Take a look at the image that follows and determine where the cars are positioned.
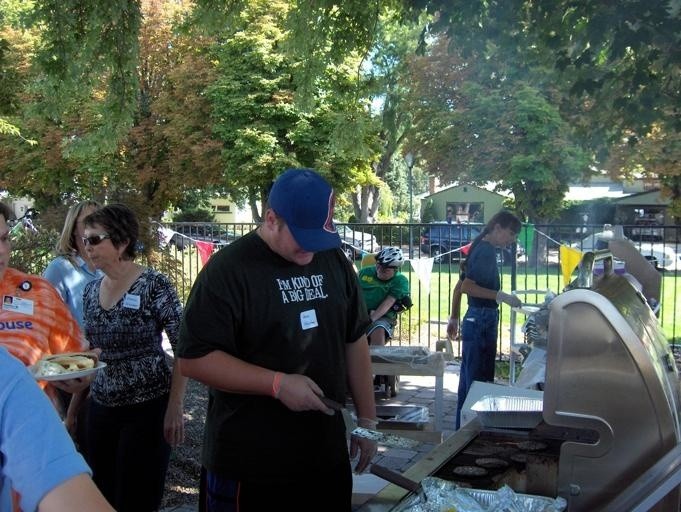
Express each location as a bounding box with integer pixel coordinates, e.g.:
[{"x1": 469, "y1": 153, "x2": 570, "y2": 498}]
[
  {"x1": 331, "y1": 220, "x2": 380, "y2": 261},
  {"x1": 629, "y1": 218, "x2": 664, "y2": 240},
  {"x1": 559, "y1": 232, "x2": 675, "y2": 269},
  {"x1": 162, "y1": 220, "x2": 241, "y2": 249}
]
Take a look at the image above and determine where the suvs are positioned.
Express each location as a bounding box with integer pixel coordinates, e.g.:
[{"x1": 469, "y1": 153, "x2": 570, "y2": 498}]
[{"x1": 419, "y1": 221, "x2": 523, "y2": 265}]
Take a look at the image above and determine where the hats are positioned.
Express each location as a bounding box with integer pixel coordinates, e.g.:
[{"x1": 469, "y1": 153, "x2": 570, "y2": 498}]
[{"x1": 267, "y1": 168, "x2": 343, "y2": 253}]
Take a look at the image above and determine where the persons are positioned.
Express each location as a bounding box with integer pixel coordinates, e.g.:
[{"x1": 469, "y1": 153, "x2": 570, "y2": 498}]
[
  {"x1": 179, "y1": 167, "x2": 382, "y2": 512},
  {"x1": 63, "y1": 204, "x2": 190, "y2": 512},
  {"x1": 39, "y1": 199, "x2": 103, "y2": 423},
  {"x1": 0, "y1": 344, "x2": 116, "y2": 512},
  {"x1": 357, "y1": 246, "x2": 411, "y2": 391},
  {"x1": 447, "y1": 210, "x2": 522, "y2": 432},
  {"x1": 0, "y1": 198, "x2": 104, "y2": 396}
]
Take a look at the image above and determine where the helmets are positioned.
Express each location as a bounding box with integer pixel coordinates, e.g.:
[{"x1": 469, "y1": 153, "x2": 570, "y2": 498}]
[{"x1": 374, "y1": 248, "x2": 404, "y2": 269}]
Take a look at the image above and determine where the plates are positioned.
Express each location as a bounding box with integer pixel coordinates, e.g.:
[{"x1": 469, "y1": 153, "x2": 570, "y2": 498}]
[{"x1": 27, "y1": 360, "x2": 108, "y2": 382}]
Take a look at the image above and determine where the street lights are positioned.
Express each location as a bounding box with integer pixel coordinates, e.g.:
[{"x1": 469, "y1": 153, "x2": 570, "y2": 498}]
[{"x1": 404, "y1": 152, "x2": 416, "y2": 260}]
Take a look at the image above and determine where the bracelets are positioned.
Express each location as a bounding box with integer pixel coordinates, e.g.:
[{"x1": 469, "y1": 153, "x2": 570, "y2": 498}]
[
  {"x1": 357, "y1": 415, "x2": 380, "y2": 426},
  {"x1": 271, "y1": 371, "x2": 283, "y2": 399}
]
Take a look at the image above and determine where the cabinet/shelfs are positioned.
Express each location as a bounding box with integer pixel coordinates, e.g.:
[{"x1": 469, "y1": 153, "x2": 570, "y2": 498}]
[{"x1": 509, "y1": 290, "x2": 557, "y2": 388}]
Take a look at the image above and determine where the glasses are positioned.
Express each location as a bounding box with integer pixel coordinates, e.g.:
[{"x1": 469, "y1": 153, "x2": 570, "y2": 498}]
[{"x1": 80, "y1": 233, "x2": 113, "y2": 246}]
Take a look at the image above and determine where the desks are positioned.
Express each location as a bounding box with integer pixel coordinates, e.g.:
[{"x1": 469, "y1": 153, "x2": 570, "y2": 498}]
[{"x1": 367, "y1": 338, "x2": 455, "y2": 435}]
